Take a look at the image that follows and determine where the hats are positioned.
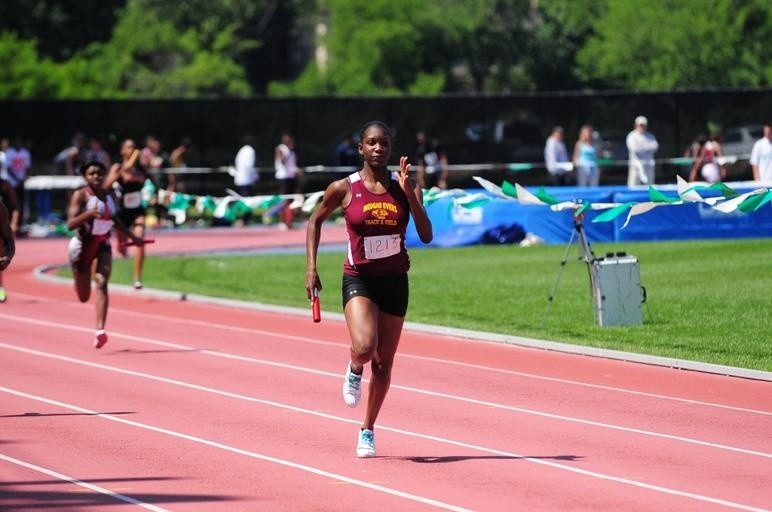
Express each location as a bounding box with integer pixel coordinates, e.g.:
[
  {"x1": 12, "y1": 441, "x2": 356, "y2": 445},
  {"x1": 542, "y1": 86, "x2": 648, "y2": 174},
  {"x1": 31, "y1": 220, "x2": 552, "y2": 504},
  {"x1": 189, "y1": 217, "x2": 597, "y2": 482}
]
[{"x1": 635, "y1": 115, "x2": 648, "y2": 125}]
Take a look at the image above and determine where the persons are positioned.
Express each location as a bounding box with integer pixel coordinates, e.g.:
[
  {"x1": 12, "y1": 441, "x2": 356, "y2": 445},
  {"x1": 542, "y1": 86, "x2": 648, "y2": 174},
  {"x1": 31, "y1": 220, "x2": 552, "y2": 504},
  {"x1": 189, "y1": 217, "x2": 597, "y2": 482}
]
[
  {"x1": 1, "y1": 160, "x2": 20, "y2": 302},
  {"x1": 0, "y1": 138, "x2": 190, "y2": 239},
  {"x1": 1, "y1": 201, "x2": 14, "y2": 272},
  {"x1": 66, "y1": 161, "x2": 143, "y2": 349},
  {"x1": 544, "y1": 116, "x2": 771, "y2": 186},
  {"x1": 102, "y1": 139, "x2": 158, "y2": 289},
  {"x1": 235, "y1": 130, "x2": 448, "y2": 189},
  {"x1": 304, "y1": 121, "x2": 434, "y2": 457}
]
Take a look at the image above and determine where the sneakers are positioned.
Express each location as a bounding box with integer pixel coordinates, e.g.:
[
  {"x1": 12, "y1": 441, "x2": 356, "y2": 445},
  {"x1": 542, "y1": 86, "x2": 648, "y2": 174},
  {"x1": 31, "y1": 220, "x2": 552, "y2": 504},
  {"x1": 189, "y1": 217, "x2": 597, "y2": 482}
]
[
  {"x1": 356, "y1": 427, "x2": 376, "y2": 459},
  {"x1": 134, "y1": 281, "x2": 142, "y2": 289},
  {"x1": 0, "y1": 287, "x2": 7, "y2": 302},
  {"x1": 341, "y1": 359, "x2": 363, "y2": 409},
  {"x1": 94, "y1": 330, "x2": 108, "y2": 349}
]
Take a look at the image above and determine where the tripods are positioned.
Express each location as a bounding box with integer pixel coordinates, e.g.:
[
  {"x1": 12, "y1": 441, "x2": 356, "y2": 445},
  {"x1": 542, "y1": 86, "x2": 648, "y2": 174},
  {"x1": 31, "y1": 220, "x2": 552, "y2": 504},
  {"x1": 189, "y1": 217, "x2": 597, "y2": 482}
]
[{"x1": 537, "y1": 222, "x2": 596, "y2": 331}]
